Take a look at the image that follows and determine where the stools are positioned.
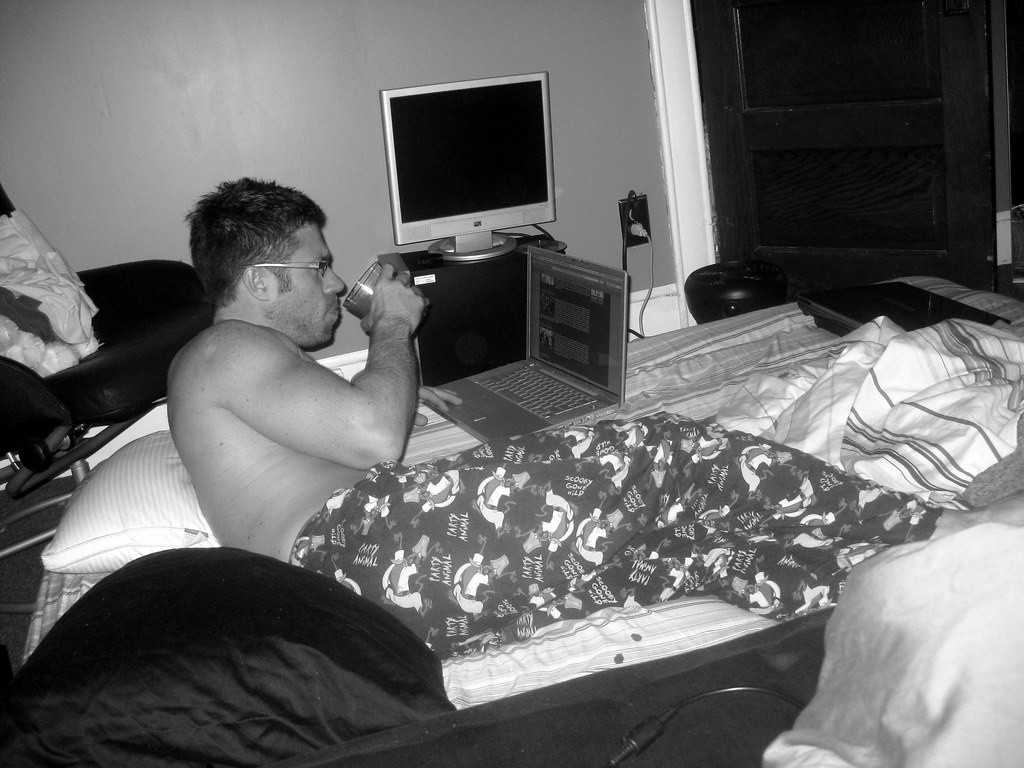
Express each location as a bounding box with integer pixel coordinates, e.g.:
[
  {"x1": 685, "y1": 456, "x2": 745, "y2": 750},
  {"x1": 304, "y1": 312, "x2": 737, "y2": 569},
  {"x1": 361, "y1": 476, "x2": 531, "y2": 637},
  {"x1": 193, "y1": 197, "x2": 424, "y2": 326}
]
[{"x1": 684, "y1": 262, "x2": 788, "y2": 324}]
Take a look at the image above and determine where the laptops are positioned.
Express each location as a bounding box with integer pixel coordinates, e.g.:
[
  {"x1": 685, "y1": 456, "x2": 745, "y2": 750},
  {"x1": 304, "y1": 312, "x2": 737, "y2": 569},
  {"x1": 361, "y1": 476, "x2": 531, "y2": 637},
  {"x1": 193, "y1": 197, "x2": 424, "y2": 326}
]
[
  {"x1": 794, "y1": 280, "x2": 1012, "y2": 337},
  {"x1": 419, "y1": 245, "x2": 631, "y2": 447}
]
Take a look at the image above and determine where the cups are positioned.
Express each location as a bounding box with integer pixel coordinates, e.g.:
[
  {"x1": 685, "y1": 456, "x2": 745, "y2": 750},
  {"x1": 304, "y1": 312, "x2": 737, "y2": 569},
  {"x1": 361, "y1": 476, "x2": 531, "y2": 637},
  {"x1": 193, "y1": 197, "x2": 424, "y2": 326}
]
[{"x1": 343, "y1": 263, "x2": 382, "y2": 319}]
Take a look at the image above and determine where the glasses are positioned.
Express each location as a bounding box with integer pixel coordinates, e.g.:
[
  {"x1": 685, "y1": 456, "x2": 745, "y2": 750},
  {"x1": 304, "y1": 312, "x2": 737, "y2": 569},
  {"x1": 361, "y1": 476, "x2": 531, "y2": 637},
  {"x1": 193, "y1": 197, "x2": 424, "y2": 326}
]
[{"x1": 230, "y1": 257, "x2": 337, "y2": 278}]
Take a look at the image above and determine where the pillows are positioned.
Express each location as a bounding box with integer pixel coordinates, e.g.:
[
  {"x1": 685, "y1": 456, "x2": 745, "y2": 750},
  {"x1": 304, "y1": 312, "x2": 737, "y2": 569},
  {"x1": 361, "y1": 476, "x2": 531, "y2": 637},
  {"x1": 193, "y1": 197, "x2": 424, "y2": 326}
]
[{"x1": 41, "y1": 432, "x2": 220, "y2": 575}]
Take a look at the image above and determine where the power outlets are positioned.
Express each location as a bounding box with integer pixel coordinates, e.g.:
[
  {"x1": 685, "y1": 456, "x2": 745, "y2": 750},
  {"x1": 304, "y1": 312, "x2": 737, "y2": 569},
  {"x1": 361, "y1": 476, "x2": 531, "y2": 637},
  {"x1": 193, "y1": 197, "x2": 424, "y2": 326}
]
[{"x1": 618, "y1": 194, "x2": 651, "y2": 248}]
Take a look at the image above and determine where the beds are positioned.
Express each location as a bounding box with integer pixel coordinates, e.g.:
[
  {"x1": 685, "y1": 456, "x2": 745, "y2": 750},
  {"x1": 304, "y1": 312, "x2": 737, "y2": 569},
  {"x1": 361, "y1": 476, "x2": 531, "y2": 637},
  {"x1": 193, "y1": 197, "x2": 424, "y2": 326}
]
[{"x1": 22, "y1": 270, "x2": 1024, "y2": 768}]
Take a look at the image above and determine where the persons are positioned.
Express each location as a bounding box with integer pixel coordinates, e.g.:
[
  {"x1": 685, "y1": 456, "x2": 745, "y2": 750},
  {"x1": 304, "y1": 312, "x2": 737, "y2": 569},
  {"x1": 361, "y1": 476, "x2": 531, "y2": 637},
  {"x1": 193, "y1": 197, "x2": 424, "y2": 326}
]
[{"x1": 167, "y1": 173, "x2": 1024, "y2": 661}]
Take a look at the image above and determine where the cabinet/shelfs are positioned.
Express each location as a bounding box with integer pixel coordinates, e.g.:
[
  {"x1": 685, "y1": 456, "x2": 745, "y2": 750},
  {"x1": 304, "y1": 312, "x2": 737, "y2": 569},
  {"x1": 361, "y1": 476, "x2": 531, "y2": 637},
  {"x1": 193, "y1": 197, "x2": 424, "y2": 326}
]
[{"x1": 378, "y1": 234, "x2": 567, "y2": 390}]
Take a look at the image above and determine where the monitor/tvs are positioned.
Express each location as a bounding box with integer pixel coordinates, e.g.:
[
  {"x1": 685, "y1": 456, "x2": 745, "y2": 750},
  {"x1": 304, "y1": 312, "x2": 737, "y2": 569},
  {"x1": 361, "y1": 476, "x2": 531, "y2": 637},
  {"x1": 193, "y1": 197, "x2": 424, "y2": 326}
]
[{"x1": 379, "y1": 70, "x2": 556, "y2": 262}]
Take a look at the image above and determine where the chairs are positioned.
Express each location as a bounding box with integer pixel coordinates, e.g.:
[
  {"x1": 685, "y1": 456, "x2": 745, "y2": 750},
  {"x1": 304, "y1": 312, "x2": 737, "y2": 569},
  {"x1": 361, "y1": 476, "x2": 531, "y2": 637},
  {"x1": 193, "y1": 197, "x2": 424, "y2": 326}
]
[{"x1": 0, "y1": 260, "x2": 213, "y2": 616}]
